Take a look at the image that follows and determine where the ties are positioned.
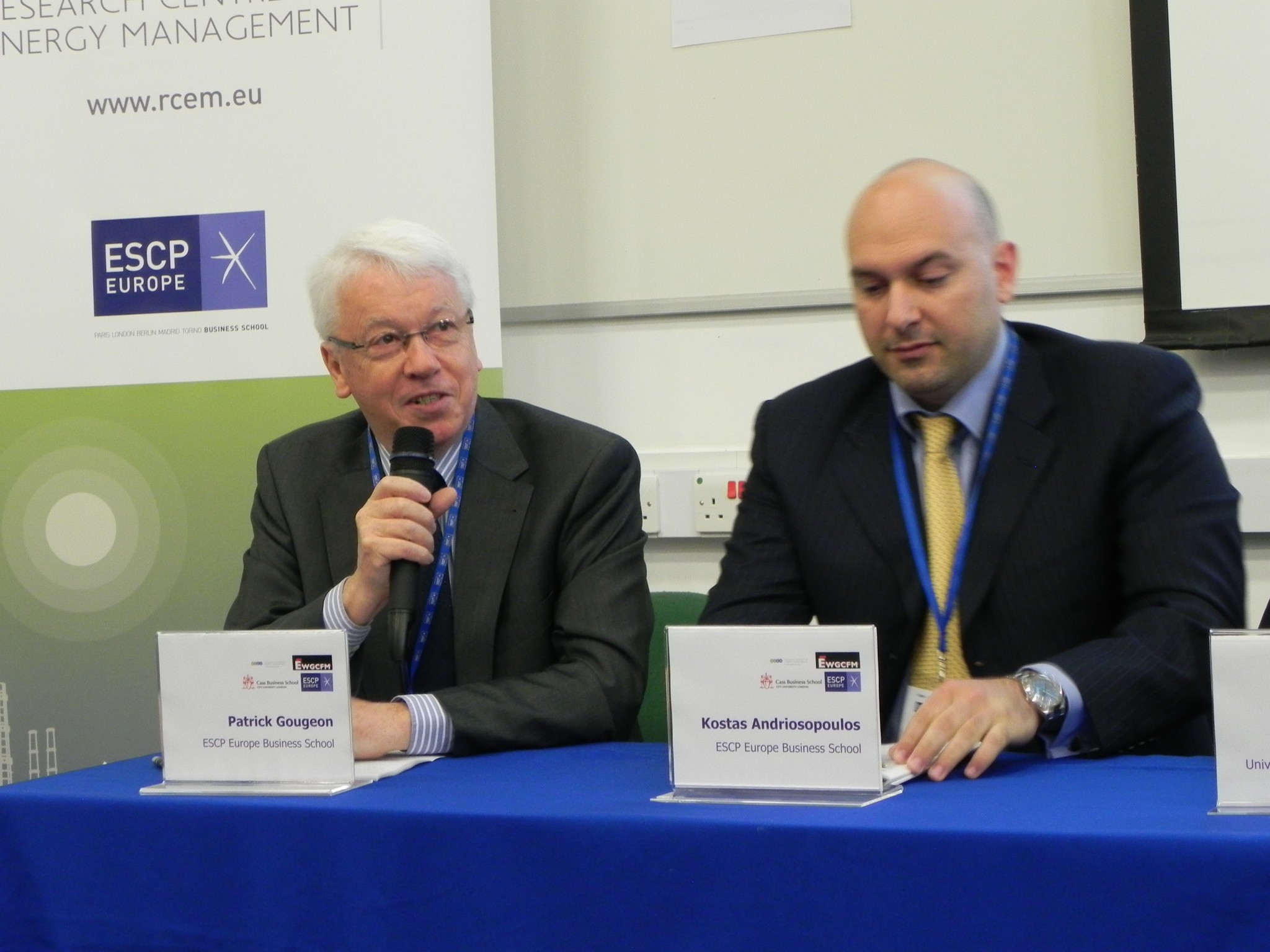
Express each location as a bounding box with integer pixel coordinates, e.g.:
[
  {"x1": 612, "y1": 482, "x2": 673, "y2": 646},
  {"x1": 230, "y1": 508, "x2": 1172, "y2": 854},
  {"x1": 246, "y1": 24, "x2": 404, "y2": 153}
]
[
  {"x1": 909, "y1": 412, "x2": 972, "y2": 690},
  {"x1": 402, "y1": 518, "x2": 453, "y2": 695}
]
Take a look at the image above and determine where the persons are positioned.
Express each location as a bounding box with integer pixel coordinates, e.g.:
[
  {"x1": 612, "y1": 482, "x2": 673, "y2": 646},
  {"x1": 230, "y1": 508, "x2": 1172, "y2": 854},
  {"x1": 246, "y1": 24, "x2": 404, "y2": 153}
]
[
  {"x1": 689, "y1": 159, "x2": 1249, "y2": 780},
  {"x1": 222, "y1": 222, "x2": 655, "y2": 758}
]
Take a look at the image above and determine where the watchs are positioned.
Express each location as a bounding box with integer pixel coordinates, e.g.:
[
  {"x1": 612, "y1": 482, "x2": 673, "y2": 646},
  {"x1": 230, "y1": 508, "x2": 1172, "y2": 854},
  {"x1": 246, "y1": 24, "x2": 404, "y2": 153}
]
[{"x1": 1008, "y1": 671, "x2": 1068, "y2": 750}]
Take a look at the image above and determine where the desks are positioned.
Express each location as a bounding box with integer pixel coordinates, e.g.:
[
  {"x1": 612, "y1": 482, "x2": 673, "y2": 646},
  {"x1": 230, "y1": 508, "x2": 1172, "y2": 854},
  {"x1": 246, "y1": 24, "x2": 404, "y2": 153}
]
[{"x1": 0, "y1": 733, "x2": 1270, "y2": 952}]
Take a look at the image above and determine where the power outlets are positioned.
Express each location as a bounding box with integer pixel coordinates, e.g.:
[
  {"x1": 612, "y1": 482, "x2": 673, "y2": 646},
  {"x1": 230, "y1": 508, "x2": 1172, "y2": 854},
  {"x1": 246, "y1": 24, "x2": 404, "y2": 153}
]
[
  {"x1": 692, "y1": 468, "x2": 751, "y2": 533},
  {"x1": 637, "y1": 470, "x2": 661, "y2": 537}
]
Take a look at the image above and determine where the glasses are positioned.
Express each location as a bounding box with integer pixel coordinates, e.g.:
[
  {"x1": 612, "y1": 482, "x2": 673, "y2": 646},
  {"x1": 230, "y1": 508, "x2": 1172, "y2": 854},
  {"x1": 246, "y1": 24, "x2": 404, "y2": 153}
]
[{"x1": 328, "y1": 308, "x2": 474, "y2": 362}]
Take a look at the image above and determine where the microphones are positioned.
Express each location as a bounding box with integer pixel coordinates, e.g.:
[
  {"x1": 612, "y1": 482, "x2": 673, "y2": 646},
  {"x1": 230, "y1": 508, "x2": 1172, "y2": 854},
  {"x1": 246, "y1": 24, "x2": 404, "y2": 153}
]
[{"x1": 390, "y1": 425, "x2": 435, "y2": 659}]
